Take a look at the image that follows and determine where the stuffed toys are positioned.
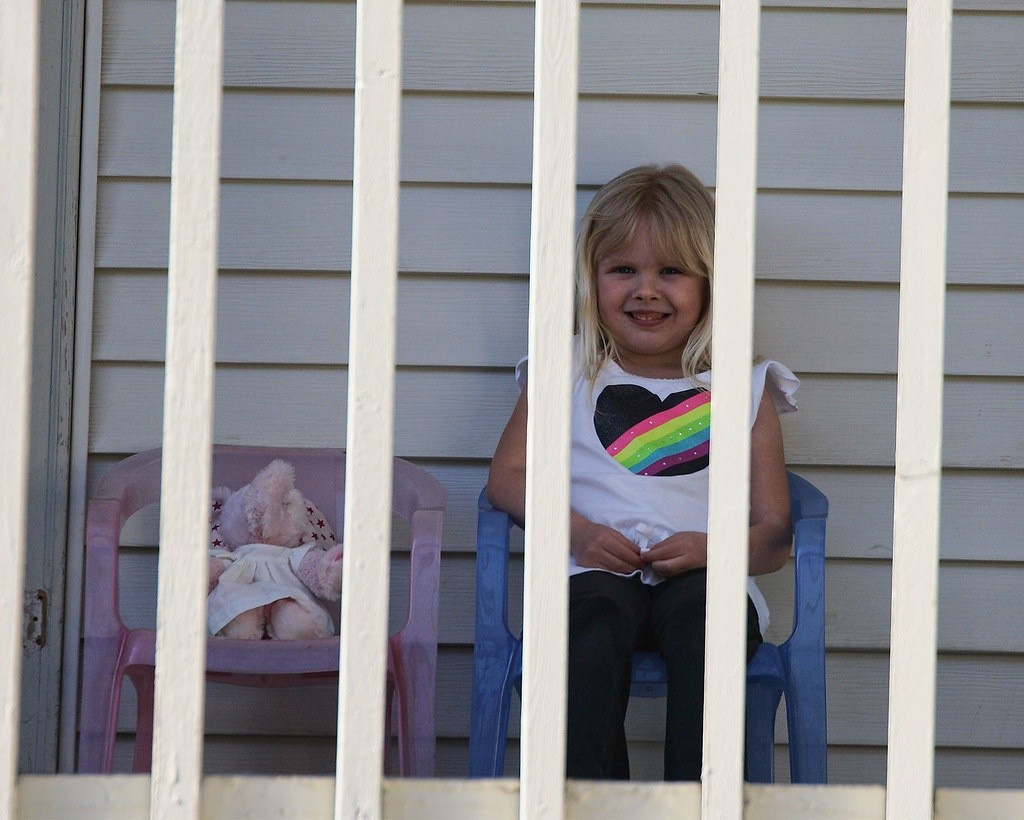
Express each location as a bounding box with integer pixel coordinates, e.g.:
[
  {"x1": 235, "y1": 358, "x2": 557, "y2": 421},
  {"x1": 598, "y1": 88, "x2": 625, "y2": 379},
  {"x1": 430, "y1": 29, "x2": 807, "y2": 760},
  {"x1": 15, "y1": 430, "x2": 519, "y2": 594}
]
[{"x1": 206, "y1": 456, "x2": 343, "y2": 641}]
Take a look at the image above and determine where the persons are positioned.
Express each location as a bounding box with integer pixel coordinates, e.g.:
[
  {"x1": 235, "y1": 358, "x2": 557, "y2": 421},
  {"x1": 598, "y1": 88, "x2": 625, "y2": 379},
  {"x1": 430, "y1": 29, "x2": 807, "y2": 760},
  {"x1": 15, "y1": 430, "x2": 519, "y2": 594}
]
[{"x1": 482, "y1": 162, "x2": 801, "y2": 781}]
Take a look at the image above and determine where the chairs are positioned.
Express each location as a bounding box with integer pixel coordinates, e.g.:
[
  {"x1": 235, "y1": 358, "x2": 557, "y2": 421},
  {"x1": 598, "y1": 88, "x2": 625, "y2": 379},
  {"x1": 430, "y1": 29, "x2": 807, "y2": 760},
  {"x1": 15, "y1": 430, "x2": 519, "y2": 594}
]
[
  {"x1": 76, "y1": 444, "x2": 447, "y2": 780},
  {"x1": 468, "y1": 467, "x2": 830, "y2": 786}
]
